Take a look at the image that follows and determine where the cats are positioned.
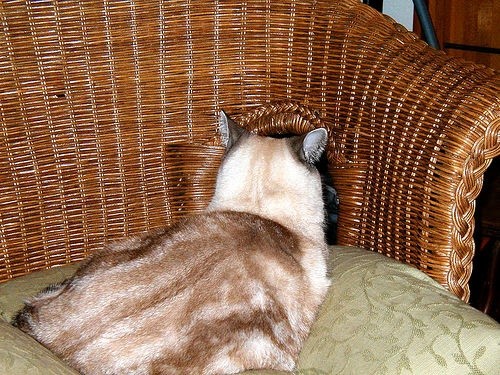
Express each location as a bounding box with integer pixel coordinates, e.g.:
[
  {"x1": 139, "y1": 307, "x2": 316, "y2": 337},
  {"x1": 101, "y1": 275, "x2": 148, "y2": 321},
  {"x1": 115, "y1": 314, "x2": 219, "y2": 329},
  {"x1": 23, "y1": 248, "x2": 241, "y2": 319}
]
[{"x1": 15, "y1": 110, "x2": 330, "y2": 375}]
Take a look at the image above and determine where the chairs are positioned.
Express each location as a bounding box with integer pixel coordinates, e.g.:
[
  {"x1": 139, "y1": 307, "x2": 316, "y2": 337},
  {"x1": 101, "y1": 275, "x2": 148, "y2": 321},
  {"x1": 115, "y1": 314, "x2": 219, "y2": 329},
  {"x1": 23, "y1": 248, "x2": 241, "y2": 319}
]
[{"x1": 0, "y1": 0, "x2": 500, "y2": 306}]
